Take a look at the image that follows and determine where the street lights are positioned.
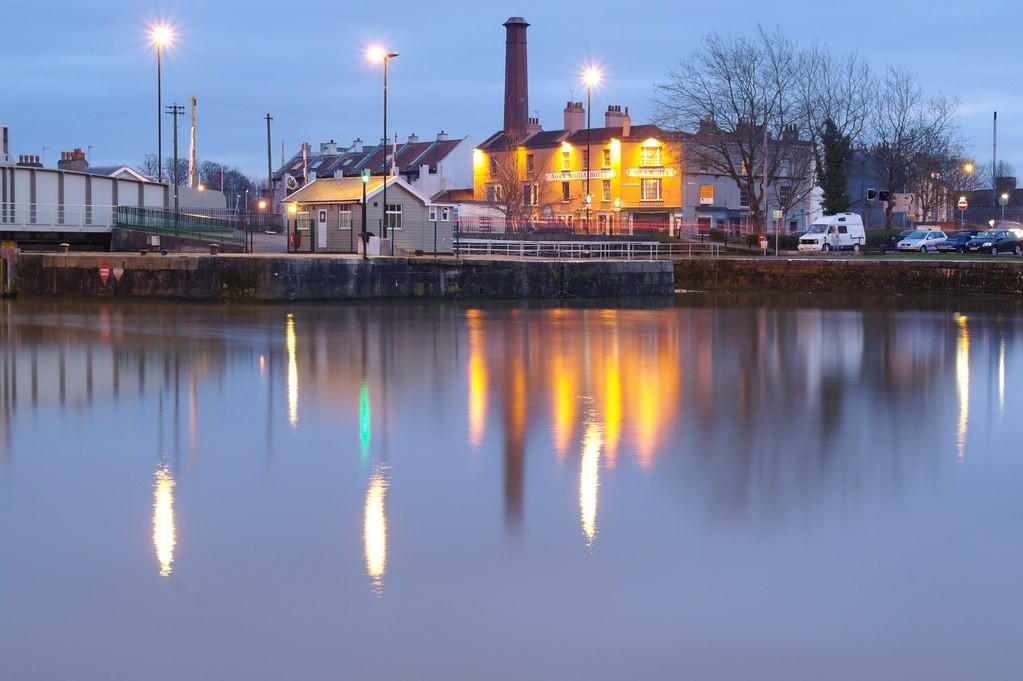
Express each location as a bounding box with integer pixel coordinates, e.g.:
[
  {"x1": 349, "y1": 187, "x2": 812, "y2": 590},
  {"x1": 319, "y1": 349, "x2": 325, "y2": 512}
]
[
  {"x1": 155, "y1": 27, "x2": 170, "y2": 181},
  {"x1": 362, "y1": 171, "x2": 367, "y2": 259},
  {"x1": 963, "y1": 163, "x2": 971, "y2": 196},
  {"x1": 369, "y1": 49, "x2": 399, "y2": 237},
  {"x1": 586, "y1": 68, "x2": 592, "y2": 235}
]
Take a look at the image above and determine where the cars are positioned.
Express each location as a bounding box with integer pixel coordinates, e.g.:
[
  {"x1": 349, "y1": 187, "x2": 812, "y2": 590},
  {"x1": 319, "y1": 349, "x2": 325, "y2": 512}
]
[
  {"x1": 959, "y1": 230, "x2": 984, "y2": 236},
  {"x1": 892, "y1": 230, "x2": 923, "y2": 249},
  {"x1": 966, "y1": 230, "x2": 1021, "y2": 256},
  {"x1": 936, "y1": 234, "x2": 975, "y2": 254},
  {"x1": 897, "y1": 231, "x2": 948, "y2": 254}
]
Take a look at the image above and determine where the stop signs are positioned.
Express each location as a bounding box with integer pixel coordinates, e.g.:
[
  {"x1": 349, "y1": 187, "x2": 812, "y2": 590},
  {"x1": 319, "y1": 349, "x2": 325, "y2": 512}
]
[{"x1": 958, "y1": 200, "x2": 968, "y2": 210}]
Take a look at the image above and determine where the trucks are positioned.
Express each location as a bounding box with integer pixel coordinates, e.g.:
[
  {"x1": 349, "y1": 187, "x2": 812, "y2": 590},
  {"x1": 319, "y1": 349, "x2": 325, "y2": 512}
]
[{"x1": 798, "y1": 212, "x2": 866, "y2": 251}]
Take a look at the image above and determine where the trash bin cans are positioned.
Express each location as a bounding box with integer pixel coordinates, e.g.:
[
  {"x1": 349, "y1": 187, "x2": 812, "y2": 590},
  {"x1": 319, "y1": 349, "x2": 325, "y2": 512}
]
[
  {"x1": 209, "y1": 244, "x2": 219, "y2": 255},
  {"x1": 880, "y1": 244, "x2": 887, "y2": 255}
]
